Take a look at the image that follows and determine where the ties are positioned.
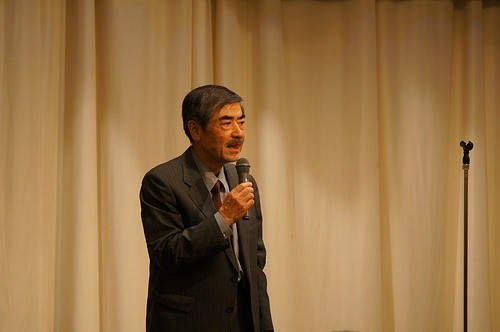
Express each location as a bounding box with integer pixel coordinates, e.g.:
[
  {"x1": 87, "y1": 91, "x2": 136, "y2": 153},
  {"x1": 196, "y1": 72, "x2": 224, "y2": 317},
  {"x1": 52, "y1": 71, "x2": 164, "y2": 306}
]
[{"x1": 209, "y1": 179, "x2": 241, "y2": 279}]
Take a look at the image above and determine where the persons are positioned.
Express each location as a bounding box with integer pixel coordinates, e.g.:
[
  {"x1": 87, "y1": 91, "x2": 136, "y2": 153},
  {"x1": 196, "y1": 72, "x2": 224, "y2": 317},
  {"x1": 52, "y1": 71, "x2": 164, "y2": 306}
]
[{"x1": 139, "y1": 85, "x2": 274, "y2": 332}]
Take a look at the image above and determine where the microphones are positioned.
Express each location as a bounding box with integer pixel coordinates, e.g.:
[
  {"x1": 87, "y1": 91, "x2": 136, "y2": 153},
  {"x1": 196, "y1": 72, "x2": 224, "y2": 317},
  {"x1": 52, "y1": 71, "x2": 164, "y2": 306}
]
[{"x1": 234, "y1": 158, "x2": 251, "y2": 219}]
